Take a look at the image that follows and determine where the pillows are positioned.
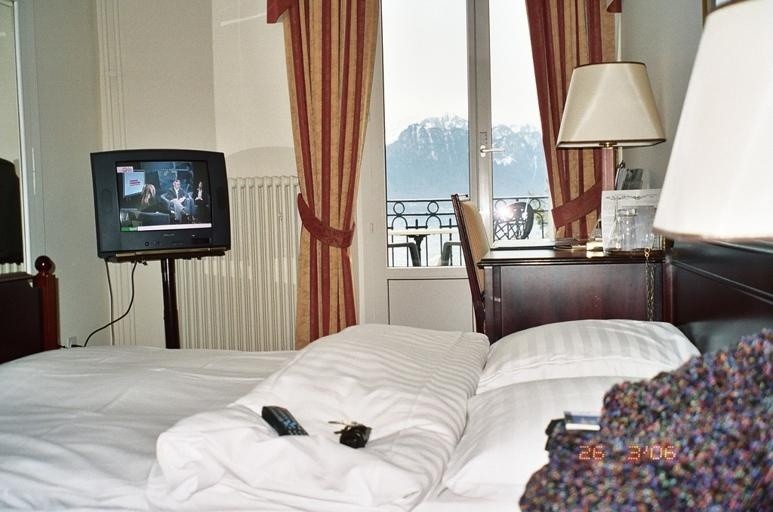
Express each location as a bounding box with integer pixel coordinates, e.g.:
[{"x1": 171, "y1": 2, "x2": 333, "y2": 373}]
[
  {"x1": 475, "y1": 317, "x2": 703, "y2": 396},
  {"x1": 439, "y1": 377, "x2": 654, "y2": 504}
]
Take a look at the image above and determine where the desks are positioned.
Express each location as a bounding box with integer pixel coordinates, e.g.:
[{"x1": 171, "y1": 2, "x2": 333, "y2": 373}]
[
  {"x1": 390, "y1": 228, "x2": 456, "y2": 266},
  {"x1": 476, "y1": 236, "x2": 664, "y2": 345}
]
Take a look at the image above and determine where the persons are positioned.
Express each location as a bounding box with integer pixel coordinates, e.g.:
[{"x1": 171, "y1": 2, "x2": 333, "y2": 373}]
[
  {"x1": 137, "y1": 184, "x2": 168, "y2": 214},
  {"x1": 191, "y1": 180, "x2": 208, "y2": 224},
  {"x1": 167, "y1": 180, "x2": 189, "y2": 224}
]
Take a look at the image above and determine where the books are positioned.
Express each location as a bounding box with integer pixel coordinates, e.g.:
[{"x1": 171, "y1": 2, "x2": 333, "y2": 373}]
[
  {"x1": 591, "y1": 169, "x2": 649, "y2": 238},
  {"x1": 601, "y1": 188, "x2": 665, "y2": 253}
]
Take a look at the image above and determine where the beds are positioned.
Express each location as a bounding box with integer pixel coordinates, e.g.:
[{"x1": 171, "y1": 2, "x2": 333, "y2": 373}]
[{"x1": 0, "y1": 238, "x2": 773, "y2": 512}]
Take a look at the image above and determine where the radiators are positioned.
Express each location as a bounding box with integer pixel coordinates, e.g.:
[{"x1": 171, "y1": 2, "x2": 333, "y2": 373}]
[{"x1": 177, "y1": 176, "x2": 302, "y2": 352}]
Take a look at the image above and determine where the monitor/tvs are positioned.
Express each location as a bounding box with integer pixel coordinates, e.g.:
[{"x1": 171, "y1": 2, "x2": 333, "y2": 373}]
[{"x1": 90, "y1": 149, "x2": 231, "y2": 263}]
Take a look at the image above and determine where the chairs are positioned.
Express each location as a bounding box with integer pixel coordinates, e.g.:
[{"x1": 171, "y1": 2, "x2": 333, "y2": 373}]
[{"x1": 439, "y1": 191, "x2": 533, "y2": 332}]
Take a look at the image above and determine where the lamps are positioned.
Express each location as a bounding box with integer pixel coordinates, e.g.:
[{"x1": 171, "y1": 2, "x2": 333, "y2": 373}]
[{"x1": 553, "y1": 60, "x2": 669, "y2": 251}]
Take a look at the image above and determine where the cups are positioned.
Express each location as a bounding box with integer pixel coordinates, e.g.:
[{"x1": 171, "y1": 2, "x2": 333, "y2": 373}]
[{"x1": 616, "y1": 214, "x2": 636, "y2": 250}]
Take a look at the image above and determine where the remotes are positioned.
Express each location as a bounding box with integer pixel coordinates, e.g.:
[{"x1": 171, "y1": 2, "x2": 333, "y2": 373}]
[{"x1": 262, "y1": 406, "x2": 308, "y2": 436}]
